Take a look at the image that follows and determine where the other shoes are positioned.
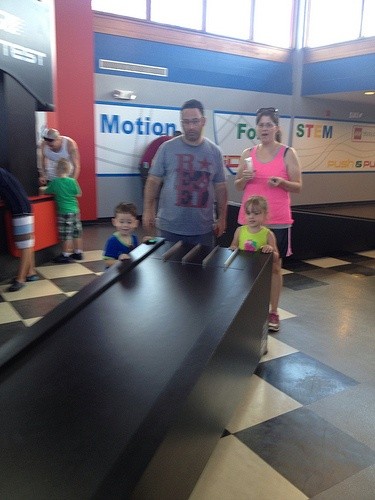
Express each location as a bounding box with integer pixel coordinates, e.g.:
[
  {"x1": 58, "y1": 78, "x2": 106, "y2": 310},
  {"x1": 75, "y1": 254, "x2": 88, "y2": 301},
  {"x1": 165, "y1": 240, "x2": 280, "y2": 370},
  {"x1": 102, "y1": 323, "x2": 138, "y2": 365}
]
[
  {"x1": 26, "y1": 275, "x2": 39, "y2": 281},
  {"x1": 7, "y1": 282, "x2": 26, "y2": 291}
]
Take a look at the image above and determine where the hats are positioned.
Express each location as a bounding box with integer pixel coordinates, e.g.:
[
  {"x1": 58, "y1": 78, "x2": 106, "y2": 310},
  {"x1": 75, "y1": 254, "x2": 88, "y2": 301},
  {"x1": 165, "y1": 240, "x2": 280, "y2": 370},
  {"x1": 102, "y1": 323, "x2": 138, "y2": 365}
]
[{"x1": 42, "y1": 128, "x2": 59, "y2": 139}]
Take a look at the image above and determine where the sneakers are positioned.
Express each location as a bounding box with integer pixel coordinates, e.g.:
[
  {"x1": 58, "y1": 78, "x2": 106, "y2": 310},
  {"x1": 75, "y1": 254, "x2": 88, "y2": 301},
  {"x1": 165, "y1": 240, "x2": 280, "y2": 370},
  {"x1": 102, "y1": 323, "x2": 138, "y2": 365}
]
[
  {"x1": 73, "y1": 253, "x2": 82, "y2": 260},
  {"x1": 55, "y1": 254, "x2": 73, "y2": 262},
  {"x1": 268, "y1": 311, "x2": 280, "y2": 331}
]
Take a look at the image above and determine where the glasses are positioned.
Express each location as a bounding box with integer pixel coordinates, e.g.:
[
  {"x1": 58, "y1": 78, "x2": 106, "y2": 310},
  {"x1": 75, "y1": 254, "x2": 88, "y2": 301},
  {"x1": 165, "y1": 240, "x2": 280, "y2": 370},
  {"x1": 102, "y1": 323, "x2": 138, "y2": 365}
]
[{"x1": 256, "y1": 107, "x2": 278, "y2": 115}]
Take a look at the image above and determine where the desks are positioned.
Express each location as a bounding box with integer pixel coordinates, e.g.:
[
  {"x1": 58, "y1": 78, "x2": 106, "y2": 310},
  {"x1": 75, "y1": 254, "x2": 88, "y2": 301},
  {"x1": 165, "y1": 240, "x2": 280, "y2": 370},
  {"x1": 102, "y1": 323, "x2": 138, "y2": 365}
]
[
  {"x1": 7, "y1": 193, "x2": 60, "y2": 257},
  {"x1": 0, "y1": 236, "x2": 274, "y2": 500}
]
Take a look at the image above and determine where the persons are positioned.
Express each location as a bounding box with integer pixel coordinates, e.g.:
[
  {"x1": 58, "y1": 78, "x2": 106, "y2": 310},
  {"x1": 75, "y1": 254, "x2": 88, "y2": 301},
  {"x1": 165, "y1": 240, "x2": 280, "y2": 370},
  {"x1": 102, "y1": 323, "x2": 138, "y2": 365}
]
[
  {"x1": 235, "y1": 107, "x2": 303, "y2": 332},
  {"x1": 229, "y1": 196, "x2": 280, "y2": 253},
  {"x1": 44, "y1": 159, "x2": 83, "y2": 263},
  {"x1": 40, "y1": 128, "x2": 80, "y2": 186},
  {"x1": 104, "y1": 203, "x2": 150, "y2": 270},
  {"x1": 139, "y1": 131, "x2": 182, "y2": 201},
  {"x1": 0, "y1": 168, "x2": 39, "y2": 291},
  {"x1": 143, "y1": 101, "x2": 229, "y2": 247}
]
